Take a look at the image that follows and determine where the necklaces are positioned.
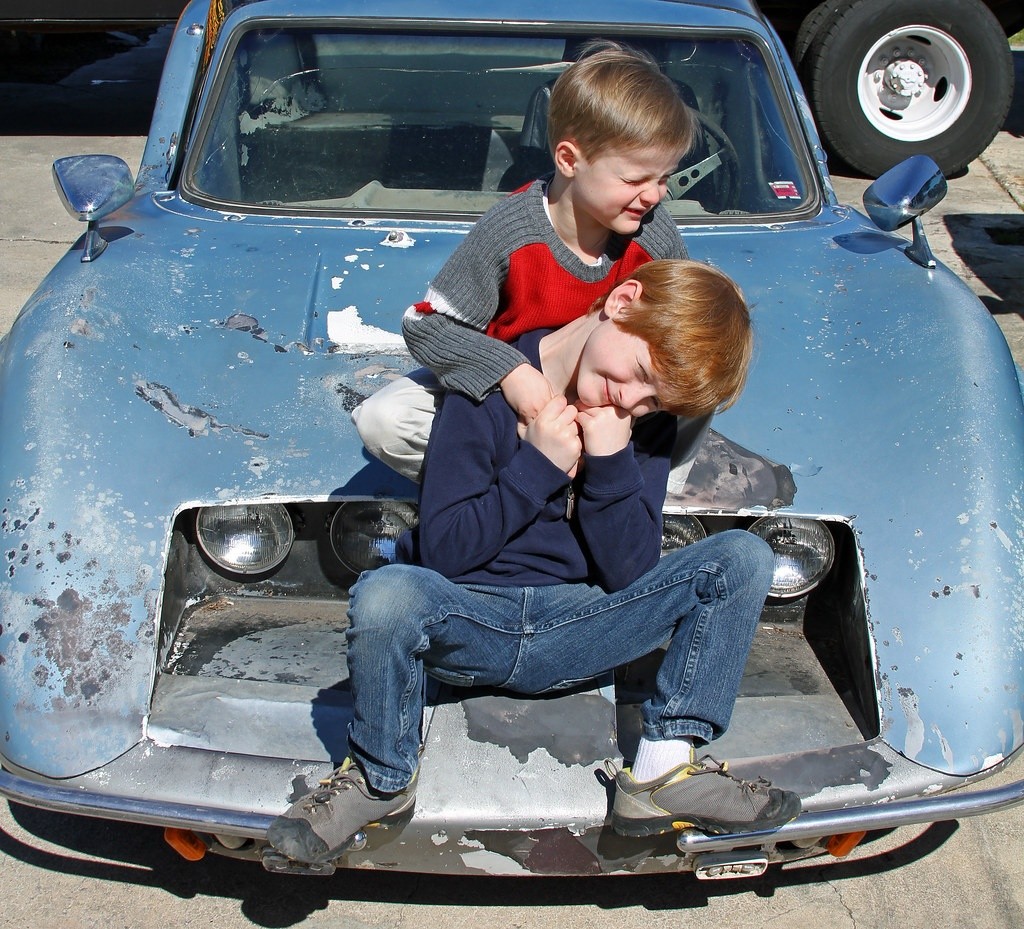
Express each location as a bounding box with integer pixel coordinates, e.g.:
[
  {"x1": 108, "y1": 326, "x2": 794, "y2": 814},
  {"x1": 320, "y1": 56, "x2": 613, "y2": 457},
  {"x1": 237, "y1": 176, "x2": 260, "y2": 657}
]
[{"x1": 567, "y1": 485, "x2": 574, "y2": 519}]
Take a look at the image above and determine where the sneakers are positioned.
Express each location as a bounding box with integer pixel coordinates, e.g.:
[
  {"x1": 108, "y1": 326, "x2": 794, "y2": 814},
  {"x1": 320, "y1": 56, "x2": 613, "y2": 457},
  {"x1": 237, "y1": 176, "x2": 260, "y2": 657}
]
[
  {"x1": 268, "y1": 756, "x2": 421, "y2": 863},
  {"x1": 604, "y1": 748, "x2": 802, "y2": 838}
]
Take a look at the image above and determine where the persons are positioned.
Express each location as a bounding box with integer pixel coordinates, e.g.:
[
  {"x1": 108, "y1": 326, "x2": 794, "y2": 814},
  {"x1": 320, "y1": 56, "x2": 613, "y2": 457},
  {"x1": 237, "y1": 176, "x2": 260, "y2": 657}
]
[
  {"x1": 265, "y1": 259, "x2": 800, "y2": 865},
  {"x1": 349, "y1": 39, "x2": 691, "y2": 487}
]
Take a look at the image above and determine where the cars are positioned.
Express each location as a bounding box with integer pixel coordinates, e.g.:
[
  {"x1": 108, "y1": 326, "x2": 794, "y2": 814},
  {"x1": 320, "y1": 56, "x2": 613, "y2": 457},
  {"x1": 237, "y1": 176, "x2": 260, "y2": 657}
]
[
  {"x1": 0, "y1": 0, "x2": 1024, "y2": 185},
  {"x1": 0, "y1": 0, "x2": 1021, "y2": 896}
]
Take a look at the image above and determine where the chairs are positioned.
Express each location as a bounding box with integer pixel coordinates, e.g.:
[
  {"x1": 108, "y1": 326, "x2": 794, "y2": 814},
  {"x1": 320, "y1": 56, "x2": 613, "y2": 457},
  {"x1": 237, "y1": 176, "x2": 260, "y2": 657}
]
[{"x1": 513, "y1": 37, "x2": 714, "y2": 209}]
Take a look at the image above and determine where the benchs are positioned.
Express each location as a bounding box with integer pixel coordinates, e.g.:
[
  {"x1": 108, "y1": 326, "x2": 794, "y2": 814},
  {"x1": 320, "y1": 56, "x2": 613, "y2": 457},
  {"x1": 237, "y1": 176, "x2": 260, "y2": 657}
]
[{"x1": 262, "y1": 55, "x2": 561, "y2": 179}]
